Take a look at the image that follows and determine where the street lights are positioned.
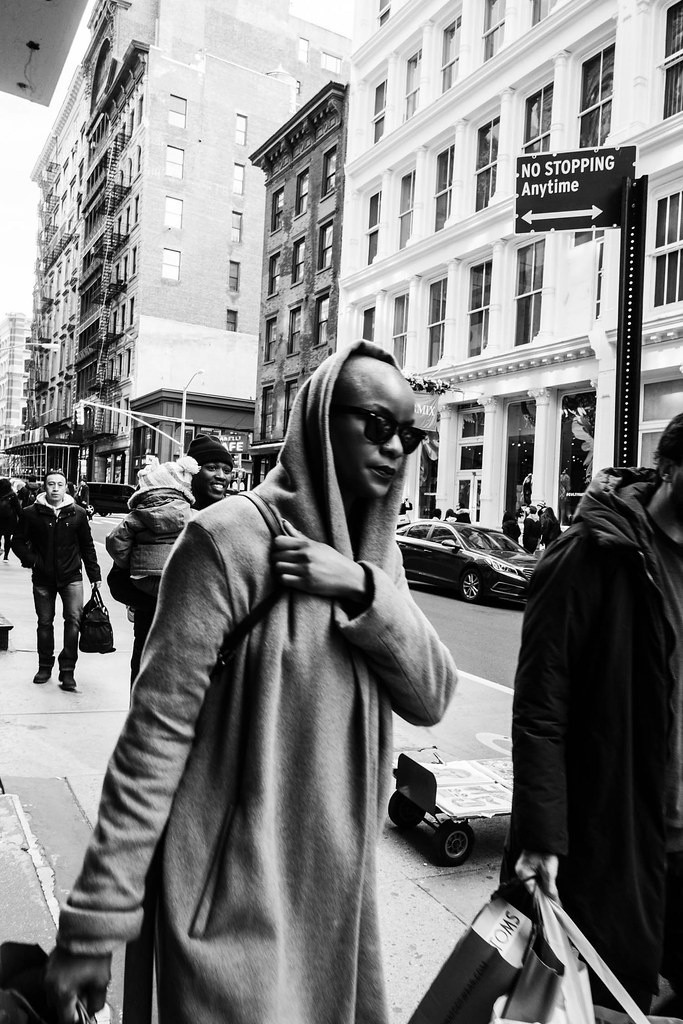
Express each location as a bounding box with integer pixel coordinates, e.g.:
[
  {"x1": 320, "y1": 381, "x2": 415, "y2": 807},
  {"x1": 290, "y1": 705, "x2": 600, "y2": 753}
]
[{"x1": 179, "y1": 370, "x2": 204, "y2": 458}]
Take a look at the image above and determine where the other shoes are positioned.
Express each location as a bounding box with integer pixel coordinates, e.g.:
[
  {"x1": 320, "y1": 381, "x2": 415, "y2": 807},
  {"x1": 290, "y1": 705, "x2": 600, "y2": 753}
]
[
  {"x1": 59, "y1": 669, "x2": 78, "y2": 688},
  {"x1": 34, "y1": 668, "x2": 54, "y2": 684}
]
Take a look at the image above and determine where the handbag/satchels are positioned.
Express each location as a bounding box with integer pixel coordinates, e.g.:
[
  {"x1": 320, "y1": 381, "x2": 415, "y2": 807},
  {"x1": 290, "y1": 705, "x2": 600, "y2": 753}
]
[
  {"x1": 404, "y1": 864, "x2": 683, "y2": 1024},
  {"x1": 79, "y1": 582, "x2": 117, "y2": 655},
  {"x1": 0, "y1": 937, "x2": 97, "y2": 1024}
]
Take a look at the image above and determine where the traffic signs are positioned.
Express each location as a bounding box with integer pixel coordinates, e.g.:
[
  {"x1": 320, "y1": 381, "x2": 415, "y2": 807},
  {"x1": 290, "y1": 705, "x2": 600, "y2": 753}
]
[{"x1": 514, "y1": 143, "x2": 637, "y2": 234}]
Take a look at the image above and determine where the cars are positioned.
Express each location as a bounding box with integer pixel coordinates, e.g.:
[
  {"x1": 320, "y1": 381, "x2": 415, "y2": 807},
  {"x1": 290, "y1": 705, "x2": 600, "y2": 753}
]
[{"x1": 395, "y1": 520, "x2": 539, "y2": 606}]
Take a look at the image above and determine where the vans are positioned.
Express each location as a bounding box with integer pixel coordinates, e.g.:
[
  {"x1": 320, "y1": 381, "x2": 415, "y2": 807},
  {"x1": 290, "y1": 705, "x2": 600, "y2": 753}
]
[{"x1": 86, "y1": 481, "x2": 136, "y2": 517}]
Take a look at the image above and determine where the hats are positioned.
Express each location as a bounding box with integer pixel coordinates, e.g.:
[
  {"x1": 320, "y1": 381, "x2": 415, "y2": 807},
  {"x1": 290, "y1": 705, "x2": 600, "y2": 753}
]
[
  {"x1": 135, "y1": 455, "x2": 201, "y2": 500},
  {"x1": 537, "y1": 500, "x2": 546, "y2": 508},
  {"x1": 186, "y1": 430, "x2": 235, "y2": 469}
]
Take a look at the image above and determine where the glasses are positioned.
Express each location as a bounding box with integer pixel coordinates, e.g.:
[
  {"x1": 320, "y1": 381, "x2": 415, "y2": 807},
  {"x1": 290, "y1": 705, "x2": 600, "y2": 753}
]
[{"x1": 334, "y1": 399, "x2": 431, "y2": 457}]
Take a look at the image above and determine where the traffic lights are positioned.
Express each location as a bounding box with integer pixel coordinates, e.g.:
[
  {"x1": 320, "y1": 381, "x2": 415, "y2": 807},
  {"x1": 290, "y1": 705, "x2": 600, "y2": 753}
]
[{"x1": 76, "y1": 407, "x2": 85, "y2": 426}]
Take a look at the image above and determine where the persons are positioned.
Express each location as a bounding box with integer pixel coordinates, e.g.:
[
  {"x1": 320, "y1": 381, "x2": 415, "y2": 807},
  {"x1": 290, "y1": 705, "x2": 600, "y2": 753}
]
[
  {"x1": 0, "y1": 475, "x2": 93, "y2": 562},
  {"x1": 10, "y1": 471, "x2": 102, "y2": 688},
  {"x1": 498, "y1": 416, "x2": 683, "y2": 1024},
  {"x1": 399, "y1": 498, "x2": 413, "y2": 516},
  {"x1": 44, "y1": 337, "x2": 458, "y2": 1024},
  {"x1": 559, "y1": 468, "x2": 573, "y2": 524},
  {"x1": 106, "y1": 434, "x2": 234, "y2": 713},
  {"x1": 432, "y1": 503, "x2": 471, "y2": 523},
  {"x1": 521, "y1": 474, "x2": 532, "y2": 507},
  {"x1": 502, "y1": 503, "x2": 562, "y2": 556}
]
